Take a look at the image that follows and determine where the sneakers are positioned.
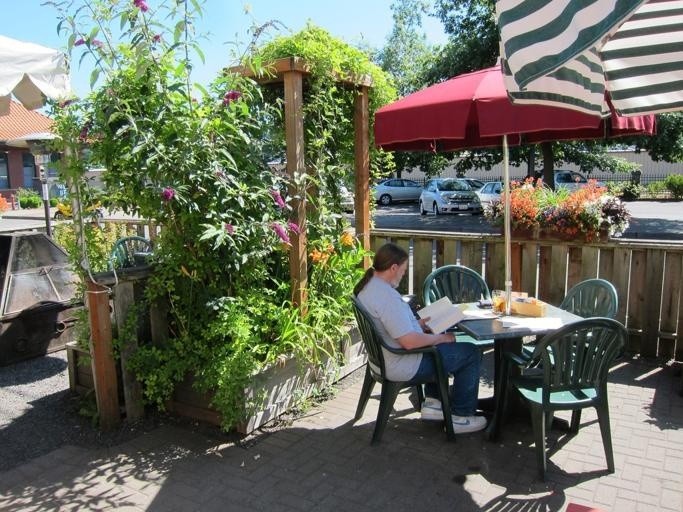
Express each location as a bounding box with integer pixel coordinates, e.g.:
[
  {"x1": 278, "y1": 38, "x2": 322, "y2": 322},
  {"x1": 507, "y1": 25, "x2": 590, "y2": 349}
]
[
  {"x1": 444, "y1": 414, "x2": 487, "y2": 434},
  {"x1": 420, "y1": 403, "x2": 445, "y2": 422}
]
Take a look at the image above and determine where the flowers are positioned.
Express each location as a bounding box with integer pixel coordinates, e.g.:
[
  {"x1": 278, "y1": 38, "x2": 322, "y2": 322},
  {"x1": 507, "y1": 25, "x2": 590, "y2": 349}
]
[{"x1": 479, "y1": 176, "x2": 630, "y2": 244}]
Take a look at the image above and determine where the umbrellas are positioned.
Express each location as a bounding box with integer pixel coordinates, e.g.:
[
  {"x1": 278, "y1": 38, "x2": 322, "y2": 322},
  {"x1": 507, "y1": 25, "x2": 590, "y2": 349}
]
[
  {"x1": 371, "y1": 58, "x2": 657, "y2": 317},
  {"x1": 0, "y1": 32, "x2": 75, "y2": 119},
  {"x1": 495, "y1": 0, "x2": 682, "y2": 119}
]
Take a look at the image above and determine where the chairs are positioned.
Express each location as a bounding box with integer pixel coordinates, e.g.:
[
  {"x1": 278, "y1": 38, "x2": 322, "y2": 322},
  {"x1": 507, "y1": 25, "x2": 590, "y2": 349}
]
[
  {"x1": 350, "y1": 294, "x2": 458, "y2": 444},
  {"x1": 421, "y1": 265, "x2": 620, "y2": 377},
  {"x1": 494, "y1": 317, "x2": 628, "y2": 481},
  {"x1": 107, "y1": 235, "x2": 154, "y2": 273}
]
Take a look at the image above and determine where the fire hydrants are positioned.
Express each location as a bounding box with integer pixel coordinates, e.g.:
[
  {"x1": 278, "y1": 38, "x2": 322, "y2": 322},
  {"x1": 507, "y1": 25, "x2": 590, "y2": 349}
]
[{"x1": 10, "y1": 193, "x2": 16, "y2": 211}]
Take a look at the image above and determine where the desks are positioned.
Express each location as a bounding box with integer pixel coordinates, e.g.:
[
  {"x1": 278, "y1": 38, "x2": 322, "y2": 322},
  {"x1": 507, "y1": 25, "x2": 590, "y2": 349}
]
[{"x1": 451, "y1": 297, "x2": 588, "y2": 441}]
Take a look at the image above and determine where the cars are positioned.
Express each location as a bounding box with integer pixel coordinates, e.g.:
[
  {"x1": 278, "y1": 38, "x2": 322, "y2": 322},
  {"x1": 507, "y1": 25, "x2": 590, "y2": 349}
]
[
  {"x1": 371, "y1": 170, "x2": 608, "y2": 216},
  {"x1": 340, "y1": 185, "x2": 354, "y2": 214}
]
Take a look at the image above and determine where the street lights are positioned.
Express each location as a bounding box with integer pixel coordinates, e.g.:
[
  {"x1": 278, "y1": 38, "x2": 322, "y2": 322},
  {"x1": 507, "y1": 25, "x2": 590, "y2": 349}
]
[{"x1": 5, "y1": 131, "x2": 63, "y2": 238}]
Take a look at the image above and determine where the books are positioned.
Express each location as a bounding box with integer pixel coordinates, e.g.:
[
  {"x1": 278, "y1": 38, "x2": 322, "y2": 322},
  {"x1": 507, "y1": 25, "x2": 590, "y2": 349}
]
[
  {"x1": 416, "y1": 295, "x2": 464, "y2": 336},
  {"x1": 455, "y1": 302, "x2": 498, "y2": 321}
]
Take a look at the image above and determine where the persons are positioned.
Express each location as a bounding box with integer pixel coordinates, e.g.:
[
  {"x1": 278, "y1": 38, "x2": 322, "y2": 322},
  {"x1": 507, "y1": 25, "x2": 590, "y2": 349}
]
[{"x1": 352, "y1": 243, "x2": 487, "y2": 436}]
